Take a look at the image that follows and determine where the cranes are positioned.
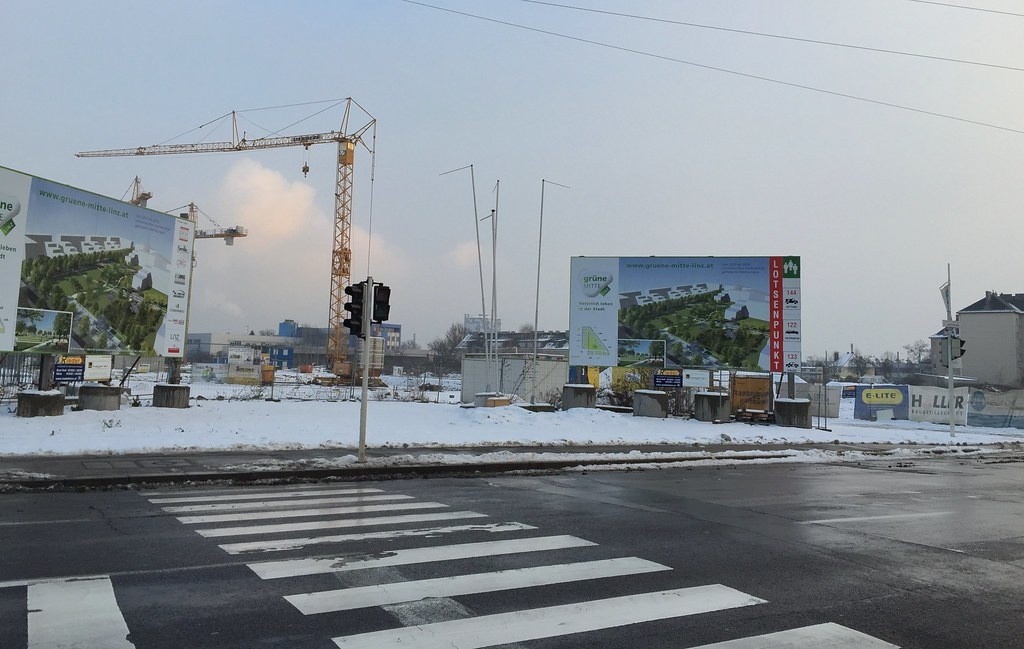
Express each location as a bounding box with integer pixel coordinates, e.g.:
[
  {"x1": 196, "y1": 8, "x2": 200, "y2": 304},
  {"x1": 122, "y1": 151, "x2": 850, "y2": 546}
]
[
  {"x1": 74, "y1": 96, "x2": 377, "y2": 378},
  {"x1": 120, "y1": 174, "x2": 152, "y2": 209},
  {"x1": 159, "y1": 201, "x2": 247, "y2": 365}
]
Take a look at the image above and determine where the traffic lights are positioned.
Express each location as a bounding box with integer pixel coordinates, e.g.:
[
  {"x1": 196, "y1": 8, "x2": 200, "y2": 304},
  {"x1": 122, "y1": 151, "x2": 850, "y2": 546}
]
[
  {"x1": 343, "y1": 285, "x2": 363, "y2": 337},
  {"x1": 951, "y1": 338, "x2": 967, "y2": 360}
]
